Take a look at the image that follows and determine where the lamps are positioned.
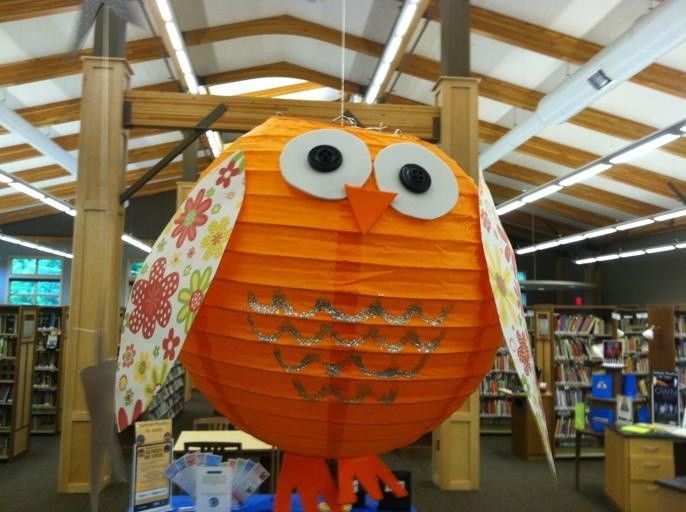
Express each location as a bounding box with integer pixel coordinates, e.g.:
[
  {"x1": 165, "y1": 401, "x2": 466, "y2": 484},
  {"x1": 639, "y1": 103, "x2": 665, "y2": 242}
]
[
  {"x1": 0, "y1": 170, "x2": 153, "y2": 263},
  {"x1": 494, "y1": 115, "x2": 686, "y2": 267}
]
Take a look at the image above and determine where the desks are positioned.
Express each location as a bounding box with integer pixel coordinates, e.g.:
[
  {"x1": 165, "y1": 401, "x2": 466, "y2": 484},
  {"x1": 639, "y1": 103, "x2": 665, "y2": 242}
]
[{"x1": 172, "y1": 429, "x2": 284, "y2": 471}]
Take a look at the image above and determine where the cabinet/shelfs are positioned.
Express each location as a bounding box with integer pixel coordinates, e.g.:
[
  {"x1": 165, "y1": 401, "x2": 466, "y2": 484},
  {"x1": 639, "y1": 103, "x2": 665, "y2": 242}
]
[
  {"x1": 141, "y1": 363, "x2": 192, "y2": 424},
  {"x1": 603, "y1": 427, "x2": 676, "y2": 512},
  {"x1": 479, "y1": 302, "x2": 686, "y2": 462},
  {"x1": 0, "y1": 304, "x2": 65, "y2": 462}
]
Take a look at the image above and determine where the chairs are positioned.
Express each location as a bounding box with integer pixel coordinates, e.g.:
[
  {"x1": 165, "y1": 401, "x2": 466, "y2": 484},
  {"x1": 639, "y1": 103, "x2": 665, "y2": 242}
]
[{"x1": 184, "y1": 416, "x2": 243, "y2": 460}]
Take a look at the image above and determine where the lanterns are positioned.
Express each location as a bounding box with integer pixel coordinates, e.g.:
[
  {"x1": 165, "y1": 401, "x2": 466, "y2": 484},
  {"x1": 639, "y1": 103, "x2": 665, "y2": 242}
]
[{"x1": 113, "y1": 113, "x2": 561, "y2": 512}]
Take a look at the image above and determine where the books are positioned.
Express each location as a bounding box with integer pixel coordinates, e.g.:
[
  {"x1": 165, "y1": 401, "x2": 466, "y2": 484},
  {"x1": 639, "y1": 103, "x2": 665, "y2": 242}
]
[
  {"x1": 0, "y1": 311, "x2": 22, "y2": 462},
  {"x1": 27, "y1": 310, "x2": 64, "y2": 435},
  {"x1": 145, "y1": 357, "x2": 186, "y2": 422},
  {"x1": 478, "y1": 311, "x2": 534, "y2": 426},
  {"x1": 622, "y1": 312, "x2": 648, "y2": 374},
  {"x1": 673, "y1": 313, "x2": 686, "y2": 425},
  {"x1": 556, "y1": 308, "x2": 621, "y2": 447}
]
